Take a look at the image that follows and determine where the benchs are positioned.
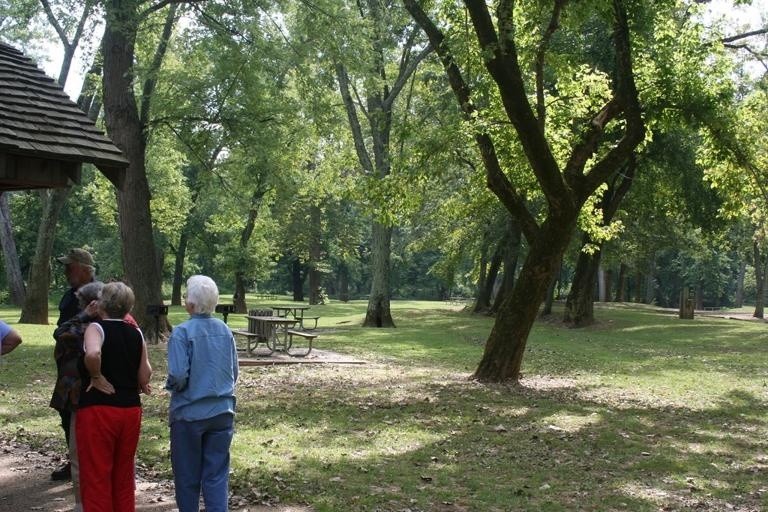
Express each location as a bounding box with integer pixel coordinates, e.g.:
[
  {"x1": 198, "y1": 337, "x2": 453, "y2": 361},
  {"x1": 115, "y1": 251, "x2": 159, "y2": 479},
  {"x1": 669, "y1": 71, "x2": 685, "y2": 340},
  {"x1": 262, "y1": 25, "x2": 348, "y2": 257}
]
[
  {"x1": 283, "y1": 329, "x2": 316, "y2": 357},
  {"x1": 229, "y1": 330, "x2": 260, "y2": 358},
  {"x1": 295, "y1": 316, "x2": 320, "y2": 330}
]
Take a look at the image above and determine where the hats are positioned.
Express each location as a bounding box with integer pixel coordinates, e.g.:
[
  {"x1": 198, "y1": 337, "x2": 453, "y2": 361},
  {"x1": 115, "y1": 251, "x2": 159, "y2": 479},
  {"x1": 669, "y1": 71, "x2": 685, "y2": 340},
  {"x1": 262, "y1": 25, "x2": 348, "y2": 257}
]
[{"x1": 56, "y1": 248, "x2": 95, "y2": 266}]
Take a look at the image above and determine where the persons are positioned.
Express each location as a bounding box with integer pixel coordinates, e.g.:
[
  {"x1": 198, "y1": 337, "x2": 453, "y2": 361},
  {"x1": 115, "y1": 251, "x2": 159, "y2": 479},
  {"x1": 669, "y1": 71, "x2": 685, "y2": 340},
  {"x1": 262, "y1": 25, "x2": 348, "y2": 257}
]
[
  {"x1": 52, "y1": 279, "x2": 106, "y2": 511},
  {"x1": 48, "y1": 247, "x2": 97, "y2": 481},
  {"x1": 161, "y1": 274, "x2": 240, "y2": 512},
  {"x1": 0, "y1": 318, "x2": 23, "y2": 357},
  {"x1": 74, "y1": 282, "x2": 153, "y2": 511}
]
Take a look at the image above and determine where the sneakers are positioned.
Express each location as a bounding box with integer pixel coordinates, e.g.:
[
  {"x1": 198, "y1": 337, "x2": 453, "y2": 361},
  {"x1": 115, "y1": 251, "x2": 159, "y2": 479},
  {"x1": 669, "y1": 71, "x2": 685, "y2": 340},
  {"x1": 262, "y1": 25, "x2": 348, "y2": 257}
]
[{"x1": 51, "y1": 462, "x2": 72, "y2": 481}]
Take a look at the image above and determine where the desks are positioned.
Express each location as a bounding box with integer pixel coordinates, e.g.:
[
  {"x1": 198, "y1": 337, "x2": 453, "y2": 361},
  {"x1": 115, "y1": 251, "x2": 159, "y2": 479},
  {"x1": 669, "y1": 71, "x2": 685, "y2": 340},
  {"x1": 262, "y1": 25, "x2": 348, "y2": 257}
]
[
  {"x1": 270, "y1": 306, "x2": 311, "y2": 329},
  {"x1": 244, "y1": 315, "x2": 300, "y2": 357}
]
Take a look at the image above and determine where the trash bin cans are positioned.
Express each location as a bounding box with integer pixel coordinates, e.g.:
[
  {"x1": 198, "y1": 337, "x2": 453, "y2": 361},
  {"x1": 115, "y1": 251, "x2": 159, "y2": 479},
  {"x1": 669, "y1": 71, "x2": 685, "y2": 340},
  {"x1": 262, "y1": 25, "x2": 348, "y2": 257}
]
[{"x1": 248, "y1": 308, "x2": 273, "y2": 342}]
